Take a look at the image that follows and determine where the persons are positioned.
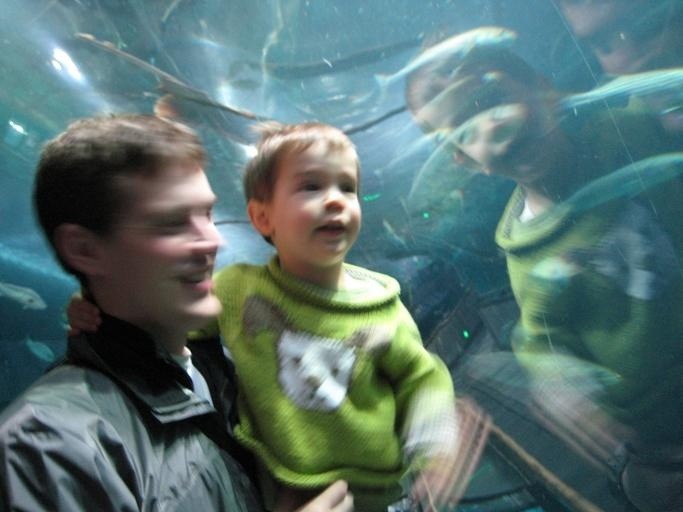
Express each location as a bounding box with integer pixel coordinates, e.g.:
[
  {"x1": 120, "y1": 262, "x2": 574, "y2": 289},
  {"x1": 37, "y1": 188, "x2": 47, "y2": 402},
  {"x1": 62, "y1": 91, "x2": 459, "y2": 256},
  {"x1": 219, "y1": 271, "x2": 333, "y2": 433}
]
[
  {"x1": 65, "y1": 120, "x2": 459, "y2": 512},
  {"x1": 0, "y1": 110, "x2": 355, "y2": 512},
  {"x1": 557, "y1": 0, "x2": 683, "y2": 130},
  {"x1": 404, "y1": 26, "x2": 683, "y2": 509}
]
